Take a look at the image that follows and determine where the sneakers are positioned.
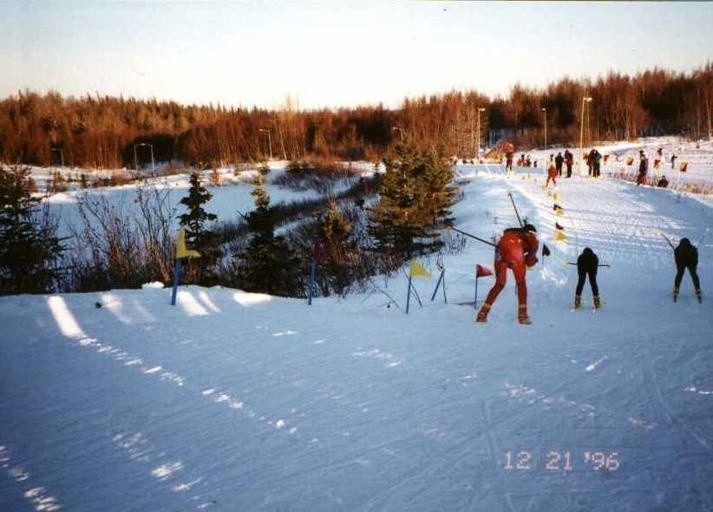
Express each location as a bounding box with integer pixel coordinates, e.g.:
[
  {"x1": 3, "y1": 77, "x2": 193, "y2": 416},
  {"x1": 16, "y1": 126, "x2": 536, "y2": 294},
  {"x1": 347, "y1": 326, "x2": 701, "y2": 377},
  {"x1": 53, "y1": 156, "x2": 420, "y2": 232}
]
[
  {"x1": 477, "y1": 318, "x2": 487, "y2": 322},
  {"x1": 519, "y1": 319, "x2": 531, "y2": 324}
]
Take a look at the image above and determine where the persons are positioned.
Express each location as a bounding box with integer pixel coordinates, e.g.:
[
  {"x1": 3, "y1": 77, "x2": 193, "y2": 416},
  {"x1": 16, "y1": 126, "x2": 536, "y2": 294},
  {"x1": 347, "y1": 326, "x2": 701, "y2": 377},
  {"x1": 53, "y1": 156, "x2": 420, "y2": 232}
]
[
  {"x1": 672, "y1": 238, "x2": 703, "y2": 304},
  {"x1": 505, "y1": 149, "x2": 602, "y2": 188},
  {"x1": 575, "y1": 247, "x2": 600, "y2": 309},
  {"x1": 475, "y1": 224, "x2": 539, "y2": 324},
  {"x1": 637, "y1": 148, "x2": 668, "y2": 187}
]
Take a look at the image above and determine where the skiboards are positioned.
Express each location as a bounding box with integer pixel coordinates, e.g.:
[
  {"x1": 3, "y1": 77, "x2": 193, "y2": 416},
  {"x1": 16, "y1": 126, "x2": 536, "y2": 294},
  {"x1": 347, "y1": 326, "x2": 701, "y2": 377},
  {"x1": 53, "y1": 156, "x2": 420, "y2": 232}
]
[
  {"x1": 673, "y1": 294, "x2": 702, "y2": 303},
  {"x1": 570, "y1": 296, "x2": 609, "y2": 315}
]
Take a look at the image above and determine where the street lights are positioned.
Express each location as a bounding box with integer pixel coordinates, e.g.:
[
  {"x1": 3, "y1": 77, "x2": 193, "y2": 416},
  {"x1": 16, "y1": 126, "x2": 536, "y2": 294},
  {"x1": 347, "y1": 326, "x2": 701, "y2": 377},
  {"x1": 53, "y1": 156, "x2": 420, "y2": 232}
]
[
  {"x1": 134, "y1": 142, "x2": 154, "y2": 171},
  {"x1": 477, "y1": 108, "x2": 487, "y2": 160},
  {"x1": 51, "y1": 148, "x2": 64, "y2": 166},
  {"x1": 256, "y1": 129, "x2": 272, "y2": 158},
  {"x1": 580, "y1": 97, "x2": 593, "y2": 148},
  {"x1": 542, "y1": 107, "x2": 547, "y2": 149},
  {"x1": 393, "y1": 127, "x2": 402, "y2": 142}
]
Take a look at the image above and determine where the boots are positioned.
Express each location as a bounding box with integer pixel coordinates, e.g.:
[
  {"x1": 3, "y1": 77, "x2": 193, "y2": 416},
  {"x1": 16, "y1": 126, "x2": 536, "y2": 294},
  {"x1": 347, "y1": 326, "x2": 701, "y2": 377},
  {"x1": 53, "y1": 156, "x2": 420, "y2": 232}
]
[
  {"x1": 594, "y1": 297, "x2": 599, "y2": 306},
  {"x1": 673, "y1": 289, "x2": 679, "y2": 297},
  {"x1": 696, "y1": 290, "x2": 702, "y2": 299},
  {"x1": 575, "y1": 296, "x2": 581, "y2": 304}
]
